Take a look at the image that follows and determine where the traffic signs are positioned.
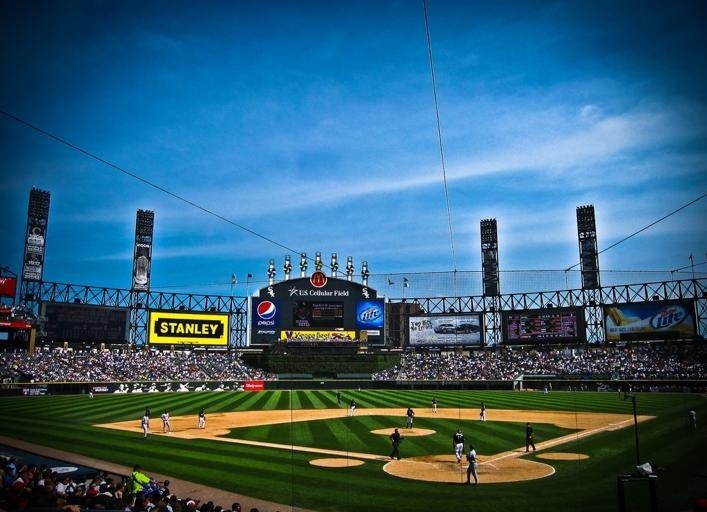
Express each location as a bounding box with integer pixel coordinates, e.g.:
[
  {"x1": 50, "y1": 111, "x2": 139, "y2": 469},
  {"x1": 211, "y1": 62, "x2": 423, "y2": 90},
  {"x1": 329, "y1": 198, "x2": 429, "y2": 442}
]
[
  {"x1": 387, "y1": 275, "x2": 410, "y2": 298},
  {"x1": 231, "y1": 272, "x2": 252, "y2": 297}
]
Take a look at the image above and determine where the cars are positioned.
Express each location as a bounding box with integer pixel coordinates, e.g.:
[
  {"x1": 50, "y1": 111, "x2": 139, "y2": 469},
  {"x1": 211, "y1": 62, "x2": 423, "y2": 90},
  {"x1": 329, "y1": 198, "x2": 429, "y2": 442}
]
[{"x1": 434, "y1": 323, "x2": 480, "y2": 334}]
